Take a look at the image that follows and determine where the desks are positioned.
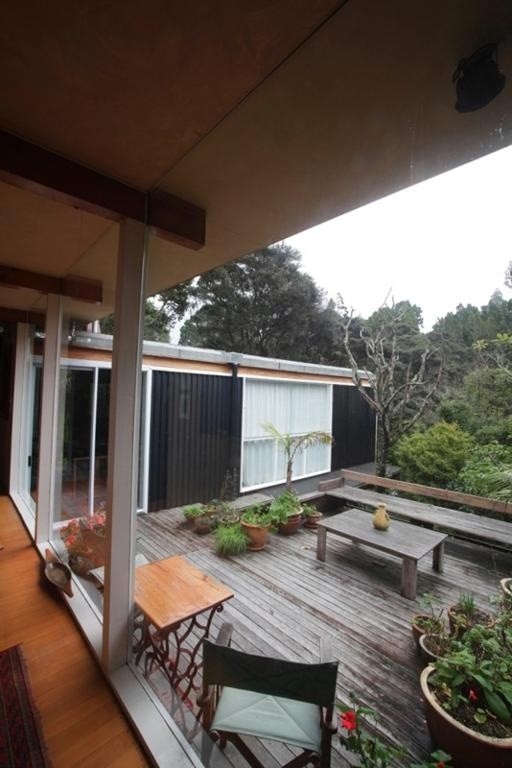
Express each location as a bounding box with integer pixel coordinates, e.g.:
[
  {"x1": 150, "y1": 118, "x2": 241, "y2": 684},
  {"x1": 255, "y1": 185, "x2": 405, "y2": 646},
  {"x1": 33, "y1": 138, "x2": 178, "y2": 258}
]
[
  {"x1": 131, "y1": 553, "x2": 234, "y2": 746},
  {"x1": 316, "y1": 508, "x2": 449, "y2": 601}
]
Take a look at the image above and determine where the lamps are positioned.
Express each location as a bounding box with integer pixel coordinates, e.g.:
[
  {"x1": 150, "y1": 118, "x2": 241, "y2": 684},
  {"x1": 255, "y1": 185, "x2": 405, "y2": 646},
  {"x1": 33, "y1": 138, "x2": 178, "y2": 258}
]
[{"x1": 452, "y1": 42, "x2": 505, "y2": 111}]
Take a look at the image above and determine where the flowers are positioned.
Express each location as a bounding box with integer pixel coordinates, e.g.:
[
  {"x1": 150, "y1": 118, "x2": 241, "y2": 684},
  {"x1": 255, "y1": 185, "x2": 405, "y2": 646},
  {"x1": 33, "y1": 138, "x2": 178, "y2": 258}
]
[
  {"x1": 426, "y1": 647, "x2": 512, "y2": 727},
  {"x1": 60, "y1": 502, "x2": 106, "y2": 560},
  {"x1": 333, "y1": 691, "x2": 455, "y2": 768}
]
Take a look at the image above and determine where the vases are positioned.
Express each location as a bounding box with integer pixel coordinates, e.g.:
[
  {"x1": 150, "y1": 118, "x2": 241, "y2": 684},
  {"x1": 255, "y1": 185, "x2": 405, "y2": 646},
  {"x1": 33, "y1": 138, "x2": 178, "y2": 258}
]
[{"x1": 419, "y1": 666, "x2": 512, "y2": 768}]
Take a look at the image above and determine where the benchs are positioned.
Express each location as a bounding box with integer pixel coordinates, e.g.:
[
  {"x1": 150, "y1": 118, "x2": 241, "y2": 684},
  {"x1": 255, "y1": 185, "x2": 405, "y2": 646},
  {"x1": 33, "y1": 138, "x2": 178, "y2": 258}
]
[{"x1": 319, "y1": 468, "x2": 512, "y2": 545}]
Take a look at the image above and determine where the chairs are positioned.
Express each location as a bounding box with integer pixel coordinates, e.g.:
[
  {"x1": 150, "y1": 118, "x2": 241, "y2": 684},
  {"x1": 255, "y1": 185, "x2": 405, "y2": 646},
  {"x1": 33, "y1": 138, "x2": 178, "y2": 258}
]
[{"x1": 197, "y1": 622, "x2": 339, "y2": 768}]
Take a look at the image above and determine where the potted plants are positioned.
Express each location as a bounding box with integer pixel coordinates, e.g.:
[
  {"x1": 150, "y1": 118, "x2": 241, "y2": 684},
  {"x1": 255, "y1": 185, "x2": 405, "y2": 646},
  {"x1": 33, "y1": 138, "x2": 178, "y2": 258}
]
[
  {"x1": 182, "y1": 433, "x2": 337, "y2": 559},
  {"x1": 410, "y1": 577, "x2": 512, "y2": 665}
]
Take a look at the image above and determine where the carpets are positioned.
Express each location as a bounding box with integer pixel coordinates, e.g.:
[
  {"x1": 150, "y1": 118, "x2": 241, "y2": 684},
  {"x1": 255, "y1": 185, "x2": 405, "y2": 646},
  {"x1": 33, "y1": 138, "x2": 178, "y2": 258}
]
[{"x1": 0, "y1": 642, "x2": 55, "y2": 768}]
